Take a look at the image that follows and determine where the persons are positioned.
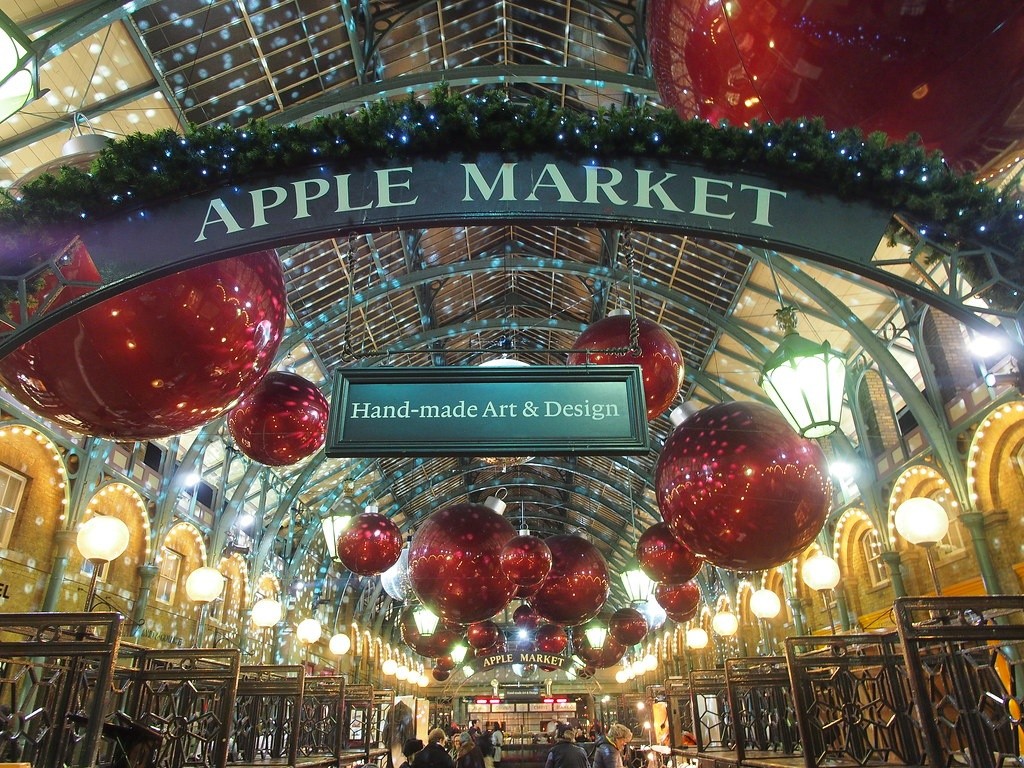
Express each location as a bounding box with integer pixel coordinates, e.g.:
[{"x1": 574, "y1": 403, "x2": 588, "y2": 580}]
[
  {"x1": 544, "y1": 719, "x2": 633, "y2": 767},
  {"x1": 400, "y1": 718, "x2": 504, "y2": 768}
]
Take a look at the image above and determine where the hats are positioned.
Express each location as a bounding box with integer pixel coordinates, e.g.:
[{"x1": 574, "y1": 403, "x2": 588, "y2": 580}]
[
  {"x1": 556, "y1": 724, "x2": 574, "y2": 739},
  {"x1": 403, "y1": 738, "x2": 423, "y2": 756}
]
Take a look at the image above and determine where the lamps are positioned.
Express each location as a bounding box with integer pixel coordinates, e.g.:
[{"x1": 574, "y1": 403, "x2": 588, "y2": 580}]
[
  {"x1": 251, "y1": 599, "x2": 282, "y2": 666},
  {"x1": 802, "y1": 554, "x2": 841, "y2": 635},
  {"x1": 750, "y1": 589, "x2": 781, "y2": 656},
  {"x1": 329, "y1": 634, "x2": 351, "y2": 675},
  {"x1": 713, "y1": 611, "x2": 738, "y2": 657},
  {"x1": 756, "y1": 247, "x2": 846, "y2": 439},
  {"x1": 687, "y1": 628, "x2": 708, "y2": 670},
  {"x1": 0, "y1": 8, "x2": 51, "y2": 124},
  {"x1": 895, "y1": 497, "x2": 949, "y2": 597},
  {"x1": 297, "y1": 619, "x2": 322, "y2": 677},
  {"x1": 565, "y1": 668, "x2": 577, "y2": 685},
  {"x1": 76, "y1": 517, "x2": 129, "y2": 612},
  {"x1": 616, "y1": 655, "x2": 659, "y2": 699},
  {"x1": 583, "y1": 615, "x2": 608, "y2": 649},
  {"x1": 382, "y1": 660, "x2": 429, "y2": 698},
  {"x1": 451, "y1": 641, "x2": 468, "y2": 665},
  {"x1": 412, "y1": 602, "x2": 440, "y2": 637},
  {"x1": 317, "y1": 458, "x2": 359, "y2": 563},
  {"x1": 185, "y1": 566, "x2": 224, "y2": 649},
  {"x1": 618, "y1": 456, "x2": 654, "y2": 603}
]
